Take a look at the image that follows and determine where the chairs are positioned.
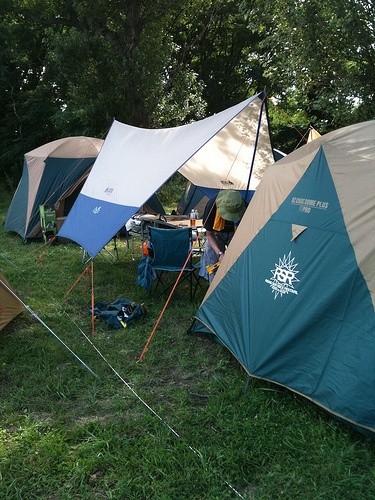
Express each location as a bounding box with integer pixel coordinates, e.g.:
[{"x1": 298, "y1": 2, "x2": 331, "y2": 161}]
[
  {"x1": 125, "y1": 218, "x2": 141, "y2": 255},
  {"x1": 148, "y1": 226, "x2": 204, "y2": 302}
]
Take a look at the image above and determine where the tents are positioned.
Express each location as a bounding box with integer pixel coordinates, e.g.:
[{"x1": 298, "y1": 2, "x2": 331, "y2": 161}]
[{"x1": 0, "y1": 92, "x2": 375, "y2": 431}]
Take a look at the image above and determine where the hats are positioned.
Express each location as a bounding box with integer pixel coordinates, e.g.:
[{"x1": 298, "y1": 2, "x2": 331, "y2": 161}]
[{"x1": 216, "y1": 188, "x2": 246, "y2": 223}]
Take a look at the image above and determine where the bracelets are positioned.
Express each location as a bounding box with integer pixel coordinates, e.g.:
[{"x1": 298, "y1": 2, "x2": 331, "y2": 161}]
[{"x1": 217, "y1": 252, "x2": 223, "y2": 258}]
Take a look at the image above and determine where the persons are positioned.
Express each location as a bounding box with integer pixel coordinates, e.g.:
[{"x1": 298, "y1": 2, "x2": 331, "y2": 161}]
[{"x1": 201, "y1": 189, "x2": 249, "y2": 285}]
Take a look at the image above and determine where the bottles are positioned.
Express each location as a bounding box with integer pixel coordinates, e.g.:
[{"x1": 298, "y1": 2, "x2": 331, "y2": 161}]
[
  {"x1": 190, "y1": 209, "x2": 195, "y2": 227},
  {"x1": 195, "y1": 209, "x2": 198, "y2": 219}
]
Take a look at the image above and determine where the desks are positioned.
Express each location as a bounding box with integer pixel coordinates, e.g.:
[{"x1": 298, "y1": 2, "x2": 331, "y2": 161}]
[{"x1": 132, "y1": 211, "x2": 204, "y2": 238}]
